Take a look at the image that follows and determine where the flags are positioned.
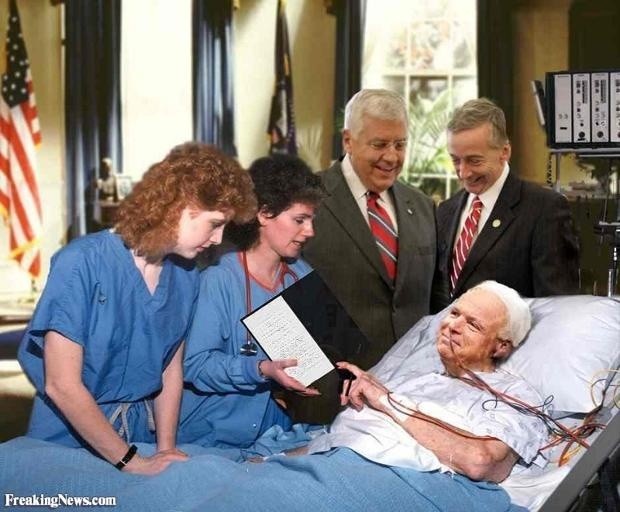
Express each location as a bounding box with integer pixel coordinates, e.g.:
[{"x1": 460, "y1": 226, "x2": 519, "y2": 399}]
[
  {"x1": 264, "y1": 0, "x2": 299, "y2": 159},
  {"x1": 1, "y1": 1, "x2": 42, "y2": 283}
]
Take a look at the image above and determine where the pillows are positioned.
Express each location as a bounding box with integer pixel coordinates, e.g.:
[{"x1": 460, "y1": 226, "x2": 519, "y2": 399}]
[{"x1": 383, "y1": 294, "x2": 619, "y2": 417}]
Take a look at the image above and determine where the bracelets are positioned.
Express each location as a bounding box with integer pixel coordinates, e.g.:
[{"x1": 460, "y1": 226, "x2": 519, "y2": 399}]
[
  {"x1": 258, "y1": 359, "x2": 266, "y2": 378},
  {"x1": 112, "y1": 444, "x2": 140, "y2": 471}
]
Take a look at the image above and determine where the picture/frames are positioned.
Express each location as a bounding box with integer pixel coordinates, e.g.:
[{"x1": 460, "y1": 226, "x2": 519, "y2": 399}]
[{"x1": 113, "y1": 173, "x2": 135, "y2": 201}]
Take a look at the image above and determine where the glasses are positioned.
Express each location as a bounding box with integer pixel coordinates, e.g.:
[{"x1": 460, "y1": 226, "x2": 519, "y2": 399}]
[{"x1": 368, "y1": 139, "x2": 408, "y2": 151}]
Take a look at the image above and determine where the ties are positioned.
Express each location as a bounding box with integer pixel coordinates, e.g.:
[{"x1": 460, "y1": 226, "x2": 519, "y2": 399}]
[
  {"x1": 366, "y1": 191, "x2": 398, "y2": 281},
  {"x1": 450, "y1": 197, "x2": 482, "y2": 297}
]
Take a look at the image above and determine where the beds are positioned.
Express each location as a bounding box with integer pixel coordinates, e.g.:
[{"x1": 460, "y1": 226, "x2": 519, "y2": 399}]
[{"x1": 1, "y1": 295, "x2": 619, "y2": 512}]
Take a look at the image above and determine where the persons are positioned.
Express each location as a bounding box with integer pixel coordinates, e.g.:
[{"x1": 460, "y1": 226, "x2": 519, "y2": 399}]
[
  {"x1": 240, "y1": 280, "x2": 550, "y2": 486},
  {"x1": 297, "y1": 88, "x2": 451, "y2": 361},
  {"x1": 18, "y1": 141, "x2": 260, "y2": 477},
  {"x1": 175, "y1": 152, "x2": 323, "y2": 448},
  {"x1": 428, "y1": 99, "x2": 584, "y2": 317},
  {"x1": 92, "y1": 158, "x2": 118, "y2": 203}
]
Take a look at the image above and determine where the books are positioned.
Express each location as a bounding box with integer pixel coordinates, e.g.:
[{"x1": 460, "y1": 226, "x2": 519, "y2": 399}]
[{"x1": 545, "y1": 69, "x2": 619, "y2": 148}]
